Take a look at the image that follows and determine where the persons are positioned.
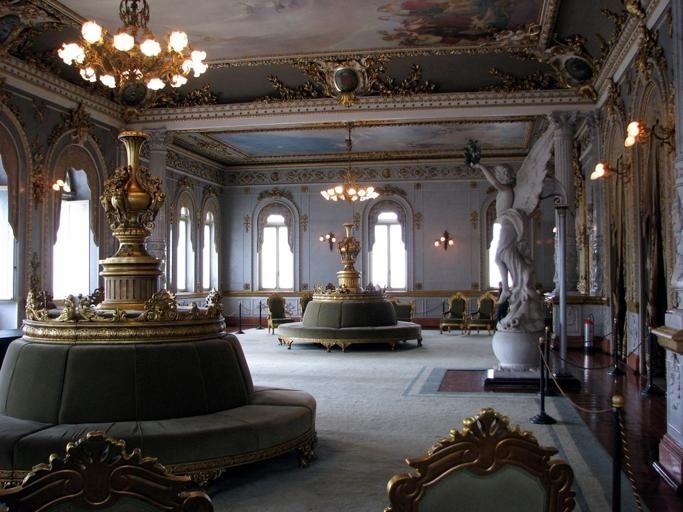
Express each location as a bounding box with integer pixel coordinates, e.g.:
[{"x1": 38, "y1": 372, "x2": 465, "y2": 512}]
[
  {"x1": 468, "y1": 161, "x2": 534, "y2": 305},
  {"x1": 493, "y1": 281, "x2": 509, "y2": 323},
  {"x1": 374, "y1": 0, "x2": 512, "y2": 47}
]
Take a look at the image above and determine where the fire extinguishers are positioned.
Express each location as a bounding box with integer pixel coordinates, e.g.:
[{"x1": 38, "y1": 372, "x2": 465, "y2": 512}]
[
  {"x1": 584, "y1": 313, "x2": 593, "y2": 353},
  {"x1": 584, "y1": 356, "x2": 590, "y2": 384}
]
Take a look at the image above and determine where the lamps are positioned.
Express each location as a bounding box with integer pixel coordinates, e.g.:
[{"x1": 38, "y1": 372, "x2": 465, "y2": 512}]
[
  {"x1": 320, "y1": 122, "x2": 380, "y2": 203},
  {"x1": 58, "y1": 0, "x2": 208, "y2": 108},
  {"x1": 319, "y1": 230, "x2": 337, "y2": 252},
  {"x1": 434, "y1": 227, "x2": 454, "y2": 251},
  {"x1": 622, "y1": 117, "x2": 675, "y2": 155},
  {"x1": 32, "y1": 179, "x2": 70, "y2": 208},
  {"x1": 589, "y1": 155, "x2": 631, "y2": 185}
]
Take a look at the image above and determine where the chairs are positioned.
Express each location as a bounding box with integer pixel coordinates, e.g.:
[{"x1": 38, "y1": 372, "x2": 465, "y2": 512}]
[
  {"x1": 439, "y1": 291, "x2": 497, "y2": 337},
  {"x1": 393, "y1": 298, "x2": 415, "y2": 319},
  {"x1": 382, "y1": 406, "x2": 578, "y2": 510},
  {"x1": 264, "y1": 290, "x2": 311, "y2": 335},
  {"x1": 0, "y1": 429, "x2": 215, "y2": 511}
]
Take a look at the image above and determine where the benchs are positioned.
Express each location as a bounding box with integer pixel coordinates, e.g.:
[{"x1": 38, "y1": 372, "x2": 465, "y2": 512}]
[
  {"x1": 0, "y1": 382, "x2": 317, "y2": 488},
  {"x1": 276, "y1": 322, "x2": 423, "y2": 353}
]
